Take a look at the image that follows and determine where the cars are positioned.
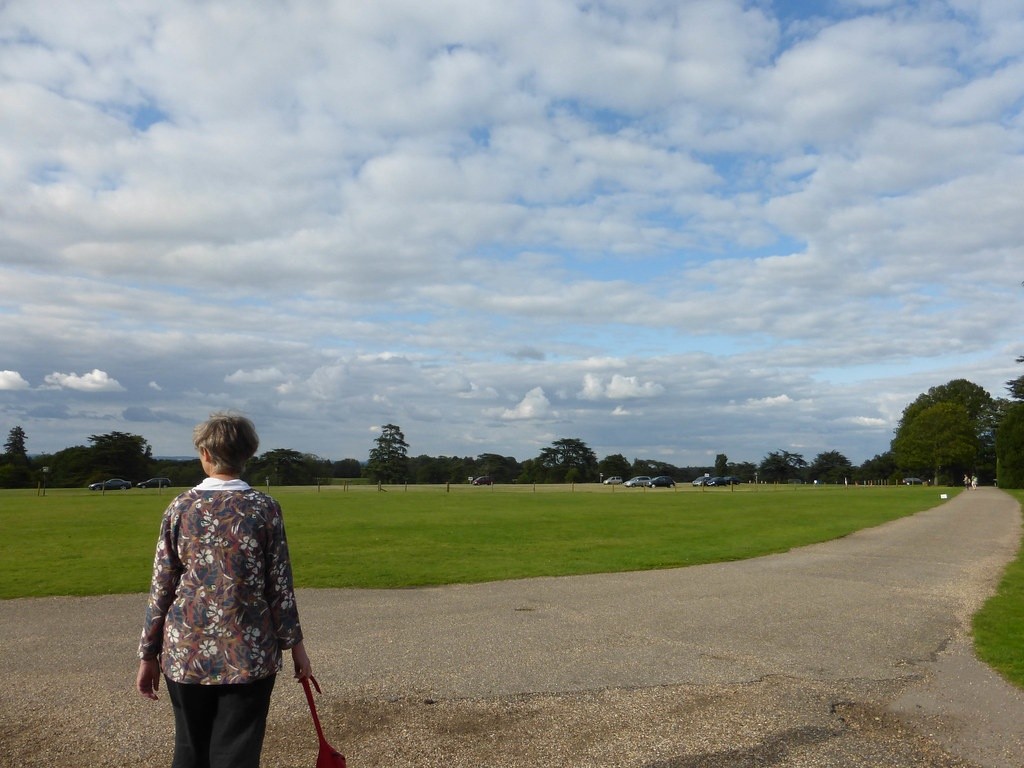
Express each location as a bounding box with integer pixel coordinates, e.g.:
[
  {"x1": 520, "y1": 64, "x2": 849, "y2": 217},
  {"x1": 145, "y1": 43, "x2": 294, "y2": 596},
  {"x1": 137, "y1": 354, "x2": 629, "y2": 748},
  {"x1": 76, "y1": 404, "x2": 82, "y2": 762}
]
[
  {"x1": 724, "y1": 478, "x2": 741, "y2": 485},
  {"x1": 137, "y1": 478, "x2": 172, "y2": 488},
  {"x1": 706, "y1": 478, "x2": 727, "y2": 487},
  {"x1": 623, "y1": 477, "x2": 651, "y2": 487},
  {"x1": 646, "y1": 477, "x2": 677, "y2": 488},
  {"x1": 603, "y1": 476, "x2": 623, "y2": 485},
  {"x1": 88, "y1": 479, "x2": 131, "y2": 490},
  {"x1": 475, "y1": 475, "x2": 496, "y2": 485},
  {"x1": 693, "y1": 477, "x2": 710, "y2": 486},
  {"x1": 902, "y1": 478, "x2": 922, "y2": 485}
]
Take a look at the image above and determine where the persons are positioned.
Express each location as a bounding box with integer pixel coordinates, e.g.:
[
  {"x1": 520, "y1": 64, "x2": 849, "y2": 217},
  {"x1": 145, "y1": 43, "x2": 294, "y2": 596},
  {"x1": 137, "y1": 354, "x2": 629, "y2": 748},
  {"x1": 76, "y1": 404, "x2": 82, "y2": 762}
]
[
  {"x1": 137, "y1": 414, "x2": 313, "y2": 768},
  {"x1": 963, "y1": 473, "x2": 977, "y2": 490}
]
